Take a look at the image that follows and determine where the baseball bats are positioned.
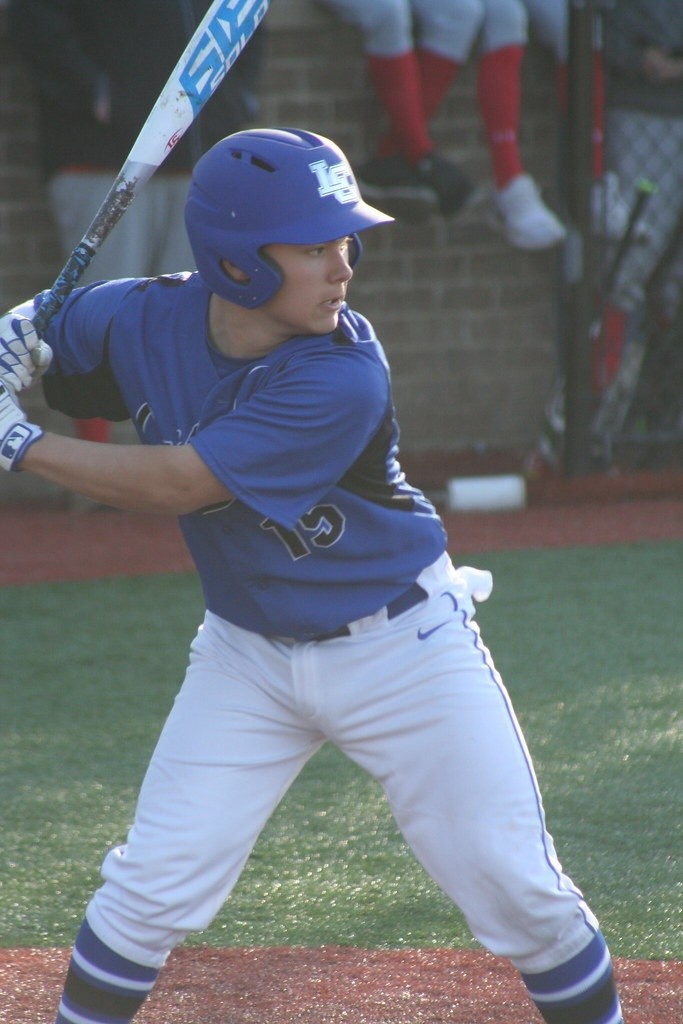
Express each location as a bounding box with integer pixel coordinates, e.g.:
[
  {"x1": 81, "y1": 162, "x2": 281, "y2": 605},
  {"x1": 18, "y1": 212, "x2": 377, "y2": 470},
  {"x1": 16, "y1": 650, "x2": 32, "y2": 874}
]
[
  {"x1": 601, "y1": 175, "x2": 683, "y2": 297},
  {"x1": 30, "y1": 0, "x2": 269, "y2": 341}
]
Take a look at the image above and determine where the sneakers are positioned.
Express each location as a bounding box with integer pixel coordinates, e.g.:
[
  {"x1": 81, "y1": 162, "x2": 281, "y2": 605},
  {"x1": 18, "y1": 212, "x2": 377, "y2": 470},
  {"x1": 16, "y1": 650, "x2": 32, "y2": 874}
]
[{"x1": 493, "y1": 175, "x2": 565, "y2": 250}]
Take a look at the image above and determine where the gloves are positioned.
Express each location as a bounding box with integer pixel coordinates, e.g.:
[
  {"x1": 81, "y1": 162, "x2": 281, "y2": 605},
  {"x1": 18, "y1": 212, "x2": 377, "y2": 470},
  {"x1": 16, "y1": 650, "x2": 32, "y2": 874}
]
[
  {"x1": 0, "y1": 312, "x2": 53, "y2": 392},
  {"x1": 0, "y1": 382, "x2": 43, "y2": 472}
]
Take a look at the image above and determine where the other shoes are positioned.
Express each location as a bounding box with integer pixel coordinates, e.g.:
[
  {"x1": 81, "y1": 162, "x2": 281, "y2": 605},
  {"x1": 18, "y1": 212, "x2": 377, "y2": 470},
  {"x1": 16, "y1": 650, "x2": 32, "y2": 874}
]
[
  {"x1": 354, "y1": 162, "x2": 422, "y2": 199},
  {"x1": 416, "y1": 155, "x2": 476, "y2": 217}
]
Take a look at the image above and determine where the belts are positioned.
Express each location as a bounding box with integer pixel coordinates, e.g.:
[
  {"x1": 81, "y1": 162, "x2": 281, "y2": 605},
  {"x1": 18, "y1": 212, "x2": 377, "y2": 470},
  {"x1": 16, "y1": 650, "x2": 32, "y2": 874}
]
[{"x1": 303, "y1": 583, "x2": 428, "y2": 641}]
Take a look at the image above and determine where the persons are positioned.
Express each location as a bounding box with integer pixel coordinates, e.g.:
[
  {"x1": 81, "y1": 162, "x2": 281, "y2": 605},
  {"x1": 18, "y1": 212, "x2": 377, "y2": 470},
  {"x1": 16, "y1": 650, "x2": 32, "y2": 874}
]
[
  {"x1": 317, "y1": 0, "x2": 654, "y2": 254},
  {"x1": 1, "y1": 127, "x2": 625, "y2": 1024}
]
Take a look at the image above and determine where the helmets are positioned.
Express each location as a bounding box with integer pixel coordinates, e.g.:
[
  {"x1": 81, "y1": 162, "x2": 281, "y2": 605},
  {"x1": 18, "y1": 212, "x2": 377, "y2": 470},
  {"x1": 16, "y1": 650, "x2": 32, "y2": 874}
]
[{"x1": 182, "y1": 127, "x2": 396, "y2": 310}]
